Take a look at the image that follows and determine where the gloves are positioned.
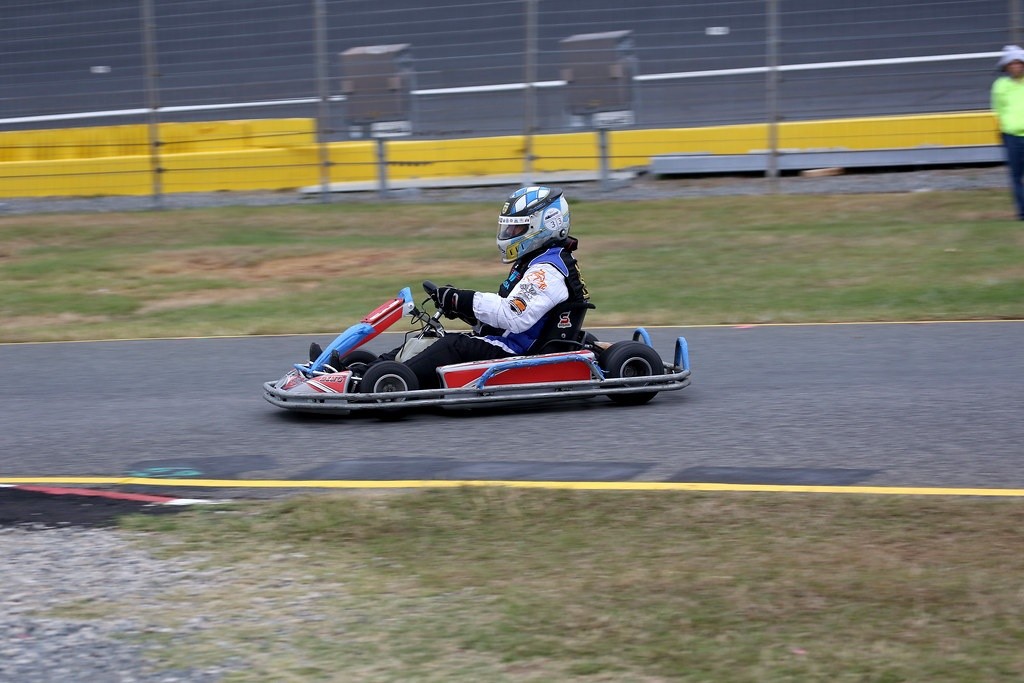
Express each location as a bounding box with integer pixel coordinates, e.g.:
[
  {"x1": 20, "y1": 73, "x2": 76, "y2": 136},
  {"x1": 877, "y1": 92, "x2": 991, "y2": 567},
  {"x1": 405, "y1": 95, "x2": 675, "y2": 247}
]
[{"x1": 431, "y1": 285, "x2": 478, "y2": 327}]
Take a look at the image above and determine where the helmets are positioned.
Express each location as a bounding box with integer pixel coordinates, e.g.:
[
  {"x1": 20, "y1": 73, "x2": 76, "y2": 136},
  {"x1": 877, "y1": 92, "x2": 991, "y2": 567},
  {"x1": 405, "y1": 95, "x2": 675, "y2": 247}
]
[{"x1": 496, "y1": 187, "x2": 571, "y2": 264}]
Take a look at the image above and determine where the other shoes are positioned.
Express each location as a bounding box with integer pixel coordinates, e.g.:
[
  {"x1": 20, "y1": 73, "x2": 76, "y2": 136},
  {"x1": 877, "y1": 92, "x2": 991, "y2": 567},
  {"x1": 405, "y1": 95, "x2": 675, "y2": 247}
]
[
  {"x1": 330, "y1": 350, "x2": 346, "y2": 373},
  {"x1": 309, "y1": 343, "x2": 323, "y2": 363}
]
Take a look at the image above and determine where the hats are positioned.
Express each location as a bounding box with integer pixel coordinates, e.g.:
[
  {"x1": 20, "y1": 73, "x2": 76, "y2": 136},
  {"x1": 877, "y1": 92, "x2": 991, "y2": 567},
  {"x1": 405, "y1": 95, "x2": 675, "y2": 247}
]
[{"x1": 997, "y1": 45, "x2": 1024, "y2": 73}]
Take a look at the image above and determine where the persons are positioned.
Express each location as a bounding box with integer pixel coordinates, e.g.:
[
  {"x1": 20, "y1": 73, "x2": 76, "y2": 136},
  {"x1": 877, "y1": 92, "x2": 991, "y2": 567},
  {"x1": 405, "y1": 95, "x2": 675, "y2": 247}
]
[
  {"x1": 309, "y1": 186, "x2": 588, "y2": 394},
  {"x1": 989, "y1": 45, "x2": 1024, "y2": 222}
]
